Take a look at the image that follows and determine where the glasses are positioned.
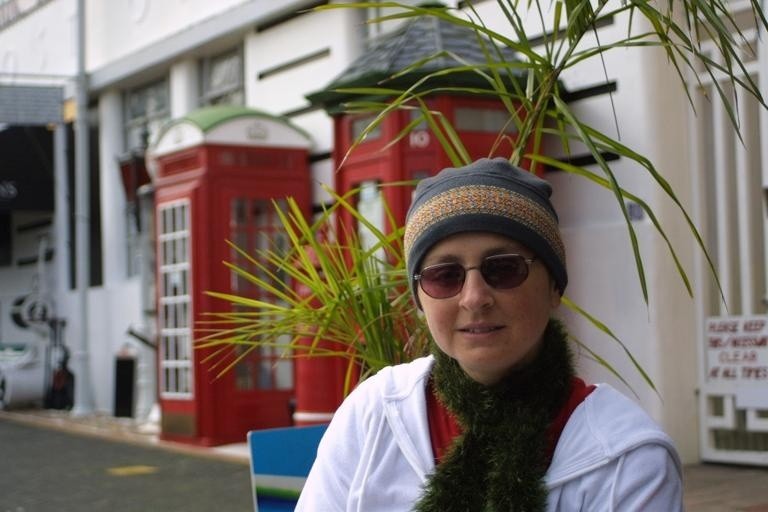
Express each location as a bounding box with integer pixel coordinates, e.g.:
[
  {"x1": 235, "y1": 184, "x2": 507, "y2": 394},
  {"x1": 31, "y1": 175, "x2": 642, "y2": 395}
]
[{"x1": 412, "y1": 251, "x2": 540, "y2": 300}]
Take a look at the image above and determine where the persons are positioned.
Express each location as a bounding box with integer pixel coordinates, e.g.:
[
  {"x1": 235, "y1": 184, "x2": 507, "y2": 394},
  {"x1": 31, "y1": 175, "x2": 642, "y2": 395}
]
[{"x1": 294, "y1": 156, "x2": 685, "y2": 511}]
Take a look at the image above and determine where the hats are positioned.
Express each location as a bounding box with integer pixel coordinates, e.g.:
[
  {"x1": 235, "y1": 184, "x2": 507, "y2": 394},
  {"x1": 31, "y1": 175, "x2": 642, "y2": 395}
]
[{"x1": 401, "y1": 155, "x2": 571, "y2": 311}]
